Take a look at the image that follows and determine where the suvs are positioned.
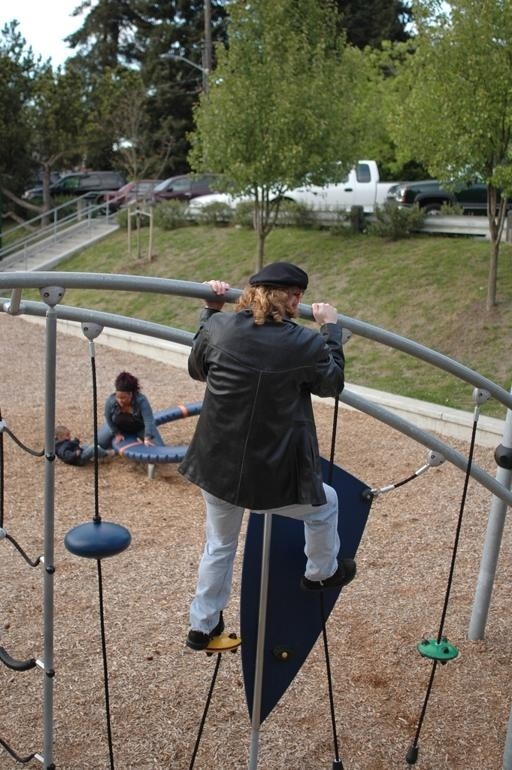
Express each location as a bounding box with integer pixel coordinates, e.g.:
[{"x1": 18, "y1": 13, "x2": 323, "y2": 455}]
[{"x1": 22, "y1": 171, "x2": 126, "y2": 205}]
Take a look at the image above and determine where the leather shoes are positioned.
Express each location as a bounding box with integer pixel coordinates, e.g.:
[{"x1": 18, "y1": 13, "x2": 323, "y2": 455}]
[
  {"x1": 298, "y1": 558, "x2": 357, "y2": 592},
  {"x1": 185, "y1": 609, "x2": 226, "y2": 651}
]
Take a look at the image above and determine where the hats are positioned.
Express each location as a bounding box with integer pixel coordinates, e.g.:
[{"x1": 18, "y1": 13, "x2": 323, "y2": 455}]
[{"x1": 249, "y1": 262, "x2": 309, "y2": 290}]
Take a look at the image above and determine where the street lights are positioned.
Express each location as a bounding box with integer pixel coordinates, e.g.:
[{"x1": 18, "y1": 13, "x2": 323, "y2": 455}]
[{"x1": 158, "y1": 51, "x2": 214, "y2": 79}]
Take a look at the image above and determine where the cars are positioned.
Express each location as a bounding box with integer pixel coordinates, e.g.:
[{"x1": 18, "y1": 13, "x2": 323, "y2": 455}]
[
  {"x1": 385, "y1": 177, "x2": 512, "y2": 217},
  {"x1": 183, "y1": 177, "x2": 283, "y2": 229},
  {"x1": 96, "y1": 172, "x2": 225, "y2": 216}
]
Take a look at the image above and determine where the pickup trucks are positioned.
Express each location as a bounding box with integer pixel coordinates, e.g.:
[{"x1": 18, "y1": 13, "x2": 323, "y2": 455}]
[{"x1": 254, "y1": 160, "x2": 401, "y2": 229}]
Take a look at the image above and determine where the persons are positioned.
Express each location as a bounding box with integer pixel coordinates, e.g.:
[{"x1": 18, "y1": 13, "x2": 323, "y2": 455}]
[
  {"x1": 175, "y1": 262, "x2": 359, "y2": 651},
  {"x1": 96, "y1": 370, "x2": 166, "y2": 448},
  {"x1": 53, "y1": 425, "x2": 116, "y2": 467}
]
